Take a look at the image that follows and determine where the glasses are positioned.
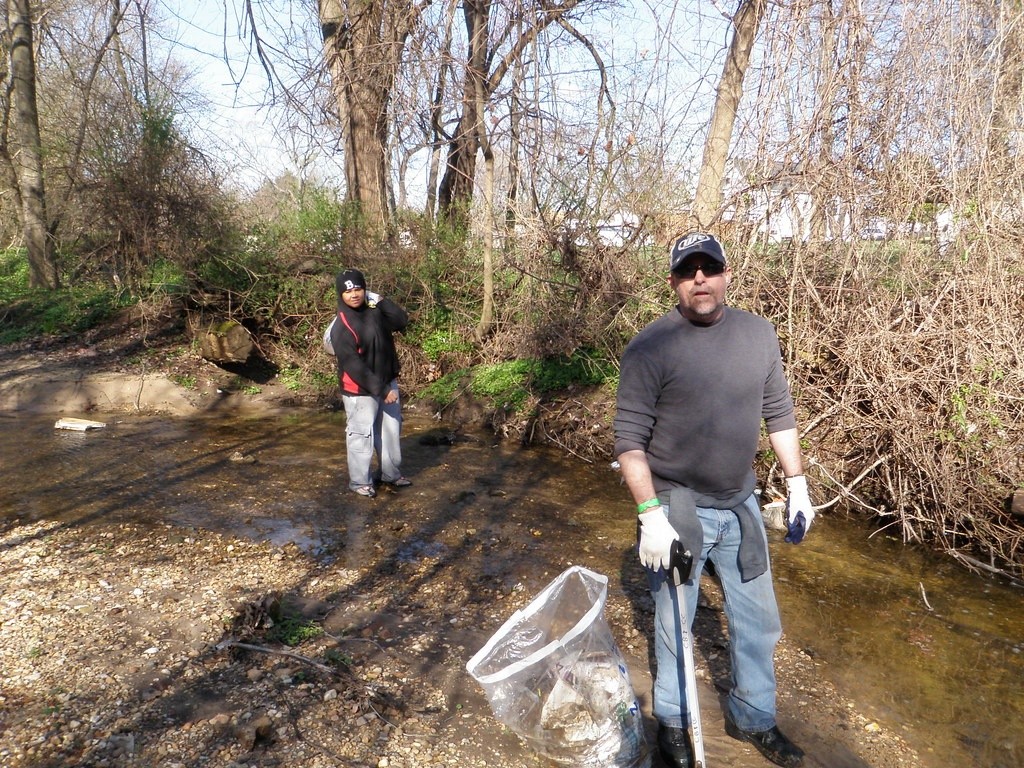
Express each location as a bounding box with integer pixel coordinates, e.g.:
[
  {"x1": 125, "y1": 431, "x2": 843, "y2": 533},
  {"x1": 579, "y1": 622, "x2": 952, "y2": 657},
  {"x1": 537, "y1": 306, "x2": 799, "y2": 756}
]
[{"x1": 672, "y1": 261, "x2": 725, "y2": 277}]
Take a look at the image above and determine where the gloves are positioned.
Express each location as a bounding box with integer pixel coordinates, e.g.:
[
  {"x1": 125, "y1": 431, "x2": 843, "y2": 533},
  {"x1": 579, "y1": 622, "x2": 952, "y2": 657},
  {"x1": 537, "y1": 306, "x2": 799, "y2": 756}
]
[
  {"x1": 637, "y1": 507, "x2": 680, "y2": 572},
  {"x1": 783, "y1": 474, "x2": 815, "y2": 545},
  {"x1": 365, "y1": 290, "x2": 383, "y2": 305}
]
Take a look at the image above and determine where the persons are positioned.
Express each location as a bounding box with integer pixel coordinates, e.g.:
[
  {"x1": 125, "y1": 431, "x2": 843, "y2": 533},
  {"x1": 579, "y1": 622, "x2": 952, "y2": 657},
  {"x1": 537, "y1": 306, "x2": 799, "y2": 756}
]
[
  {"x1": 329, "y1": 268, "x2": 413, "y2": 497},
  {"x1": 612, "y1": 232, "x2": 817, "y2": 768}
]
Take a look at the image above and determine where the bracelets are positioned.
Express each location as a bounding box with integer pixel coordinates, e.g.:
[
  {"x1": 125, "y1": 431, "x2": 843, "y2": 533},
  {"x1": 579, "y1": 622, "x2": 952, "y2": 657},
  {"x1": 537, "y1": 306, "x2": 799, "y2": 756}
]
[{"x1": 636, "y1": 498, "x2": 660, "y2": 514}]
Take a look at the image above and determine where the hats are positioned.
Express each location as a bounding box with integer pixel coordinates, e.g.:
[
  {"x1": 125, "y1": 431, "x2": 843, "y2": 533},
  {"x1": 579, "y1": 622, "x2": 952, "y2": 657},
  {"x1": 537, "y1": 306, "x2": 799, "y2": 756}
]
[
  {"x1": 336, "y1": 269, "x2": 366, "y2": 297},
  {"x1": 669, "y1": 232, "x2": 726, "y2": 270}
]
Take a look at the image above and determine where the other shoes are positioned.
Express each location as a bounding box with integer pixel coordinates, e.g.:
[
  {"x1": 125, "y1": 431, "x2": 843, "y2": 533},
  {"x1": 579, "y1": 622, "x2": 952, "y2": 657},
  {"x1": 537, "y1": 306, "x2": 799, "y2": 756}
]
[
  {"x1": 354, "y1": 485, "x2": 376, "y2": 498},
  {"x1": 387, "y1": 478, "x2": 412, "y2": 487}
]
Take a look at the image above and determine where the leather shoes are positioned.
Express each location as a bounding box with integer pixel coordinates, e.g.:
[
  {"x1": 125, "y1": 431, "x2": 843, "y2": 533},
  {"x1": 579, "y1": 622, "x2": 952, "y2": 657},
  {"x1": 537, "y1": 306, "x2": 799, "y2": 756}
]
[
  {"x1": 657, "y1": 722, "x2": 695, "y2": 768},
  {"x1": 724, "y1": 719, "x2": 806, "y2": 768}
]
